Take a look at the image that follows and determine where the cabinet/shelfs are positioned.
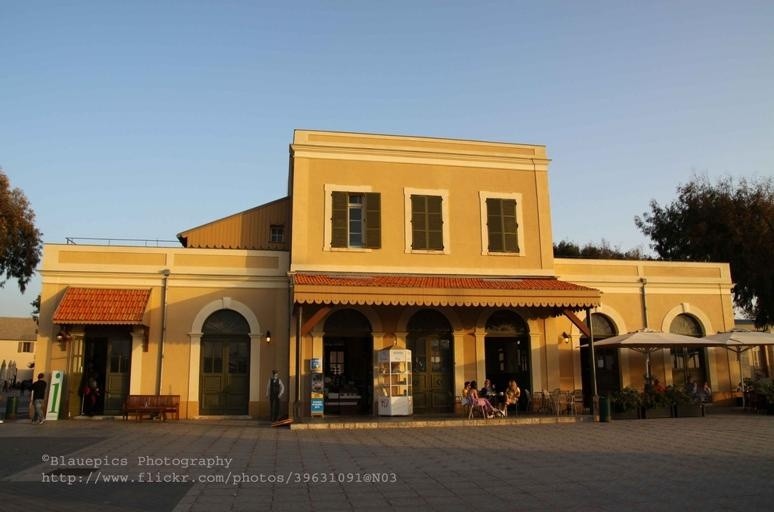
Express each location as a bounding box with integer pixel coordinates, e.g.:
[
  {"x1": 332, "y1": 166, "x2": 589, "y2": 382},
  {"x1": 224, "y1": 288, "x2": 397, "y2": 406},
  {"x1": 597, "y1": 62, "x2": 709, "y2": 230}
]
[{"x1": 377, "y1": 347, "x2": 413, "y2": 415}]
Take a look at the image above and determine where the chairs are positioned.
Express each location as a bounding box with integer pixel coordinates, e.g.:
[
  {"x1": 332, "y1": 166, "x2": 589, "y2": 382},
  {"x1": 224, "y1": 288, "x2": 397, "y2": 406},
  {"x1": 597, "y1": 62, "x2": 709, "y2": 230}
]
[{"x1": 463, "y1": 387, "x2": 585, "y2": 418}]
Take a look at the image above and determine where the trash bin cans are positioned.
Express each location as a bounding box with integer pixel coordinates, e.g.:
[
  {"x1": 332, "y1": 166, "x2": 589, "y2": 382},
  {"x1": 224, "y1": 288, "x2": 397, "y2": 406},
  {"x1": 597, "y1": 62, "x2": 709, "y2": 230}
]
[
  {"x1": 600, "y1": 397, "x2": 611, "y2": 422},
  {"x1": 5, "y1": 396, "x2": 18, "y2": 420}
]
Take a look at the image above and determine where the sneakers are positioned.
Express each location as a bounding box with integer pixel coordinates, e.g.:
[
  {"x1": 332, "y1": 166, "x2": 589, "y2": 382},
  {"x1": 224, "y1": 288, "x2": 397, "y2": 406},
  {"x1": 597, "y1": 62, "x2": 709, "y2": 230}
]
[{"x1": 488, "y1": 407, "x2": 505, "y2": 419}]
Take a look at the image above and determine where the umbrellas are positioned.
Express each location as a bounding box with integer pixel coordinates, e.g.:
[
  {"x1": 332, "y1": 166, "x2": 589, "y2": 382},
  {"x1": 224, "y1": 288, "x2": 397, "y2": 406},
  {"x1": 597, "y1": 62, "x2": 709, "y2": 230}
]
[
  {"x1": 575, "y1": 328, "x2": 726, "y2": 384},
  {"x1": 700, "y1": 328, "x2": 774, "y2": 409}
]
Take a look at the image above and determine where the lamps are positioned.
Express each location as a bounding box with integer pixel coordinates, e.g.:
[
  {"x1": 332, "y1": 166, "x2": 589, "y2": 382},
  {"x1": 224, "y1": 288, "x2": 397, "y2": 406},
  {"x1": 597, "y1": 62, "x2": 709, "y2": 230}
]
[
  {"x1": 265, "y1": 331, "x2": 271, "y2": 343},
  {"x1": 56, "y1": 332, "x2": 63, "y2": 342},
  {"x1": 563, "y1": 331, "x2": 569, "y2": 344}
]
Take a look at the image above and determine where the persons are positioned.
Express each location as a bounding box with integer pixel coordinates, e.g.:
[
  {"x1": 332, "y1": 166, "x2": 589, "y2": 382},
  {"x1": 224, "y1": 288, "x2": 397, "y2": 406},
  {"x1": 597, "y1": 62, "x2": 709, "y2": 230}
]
[
  {"x1": 684, "y1": 374, "x2": 698, "y2": 399},
  {"x1": 462, "y1": 379, "x2": 520, "y2": 419},
  {"x1": 81, "y1": 361, "x2": 102, "y2": 416},
  {"x1": 265, "y1": 369, "x2": 285, "y2": 423},
  {"x1": 699, "y1": 382, "x2": 711, "y2": 409},
  {"x1": 29, "y1": 373, "x2": 48, "y2": 424},
  {"x1": 652, "y1": 379, "x2": 668, "y2": 394}
]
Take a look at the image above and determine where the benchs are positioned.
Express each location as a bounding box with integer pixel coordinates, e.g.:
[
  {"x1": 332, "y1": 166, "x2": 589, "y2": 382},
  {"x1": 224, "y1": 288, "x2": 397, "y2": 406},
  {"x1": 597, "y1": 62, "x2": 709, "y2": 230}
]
[{"x1": 121, "y1": 393, "x2": 181, "y2": 421}]
